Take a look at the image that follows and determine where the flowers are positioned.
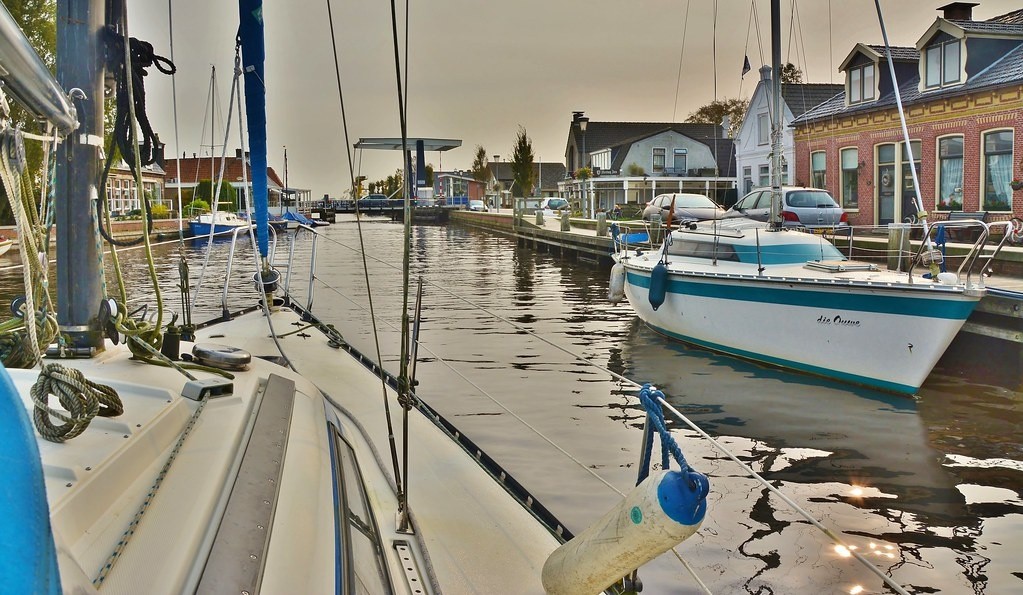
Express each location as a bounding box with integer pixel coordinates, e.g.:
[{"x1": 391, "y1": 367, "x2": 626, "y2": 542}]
[{"x1": 1009, "y1": 178, "x2": 1020, "y2": 186}]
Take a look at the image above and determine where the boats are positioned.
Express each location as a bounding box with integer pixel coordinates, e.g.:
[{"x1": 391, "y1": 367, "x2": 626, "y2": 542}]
[
  {"x1": 313, "y1": 219, "x2": 330, "y2": 227},
  {"x1": 247, "y1": 212, "x2": 289, "y2": 236}
]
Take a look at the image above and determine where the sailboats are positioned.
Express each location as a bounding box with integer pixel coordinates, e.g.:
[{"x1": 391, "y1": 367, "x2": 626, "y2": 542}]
[
  {"x1": 607, "y1": 319, "x2": 979, "y2": 594},
  {"x1": 606, "y1": 0, "x2": 1014, "y2": 396},
  {"x1": 187, "y1": 63, "x2": 248, "y2": 242},
  {"x1": 279, "y1": 146, "x2": 312, "y2": 228}
]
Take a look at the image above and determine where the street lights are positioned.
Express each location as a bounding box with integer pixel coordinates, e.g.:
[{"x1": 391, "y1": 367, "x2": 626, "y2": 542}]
[
  {"x1": 459, "y1": 170, "x2": 463, "y2": 211},
  {"x1": 577, "y1": 116, "x2": 590, "y2": 218},
  {"x1": 494, "y1": 155, "x2": 500, "y2": 213}
]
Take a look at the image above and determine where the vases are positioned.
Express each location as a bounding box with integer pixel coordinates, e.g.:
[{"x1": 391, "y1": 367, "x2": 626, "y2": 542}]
[{"x1": 1011, "y1": 184, "x2": 1023, "y2": 190}]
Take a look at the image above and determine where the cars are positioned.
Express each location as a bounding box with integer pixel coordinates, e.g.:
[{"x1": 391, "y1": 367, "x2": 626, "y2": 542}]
[
  {"x1": 466, "y1": 199, "x2": 488, "y2": 212},
  {"x1": 534, "y1": 197, "x2": 572, "y2": 217},
  {"x1": 641, "y1": 193, "x2": 725, "y2": 225},
  {"x1": 721, "y1": 186, "x2": 849, "y2": 237},
  {"x1": 352, "y1": 194, "x2": 389, "y2": 208}
]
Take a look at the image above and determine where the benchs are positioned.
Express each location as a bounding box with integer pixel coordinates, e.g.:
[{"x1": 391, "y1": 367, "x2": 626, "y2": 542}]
[{"x1": 936, "y1": 212, "x2": 989, "y2": 240}]
